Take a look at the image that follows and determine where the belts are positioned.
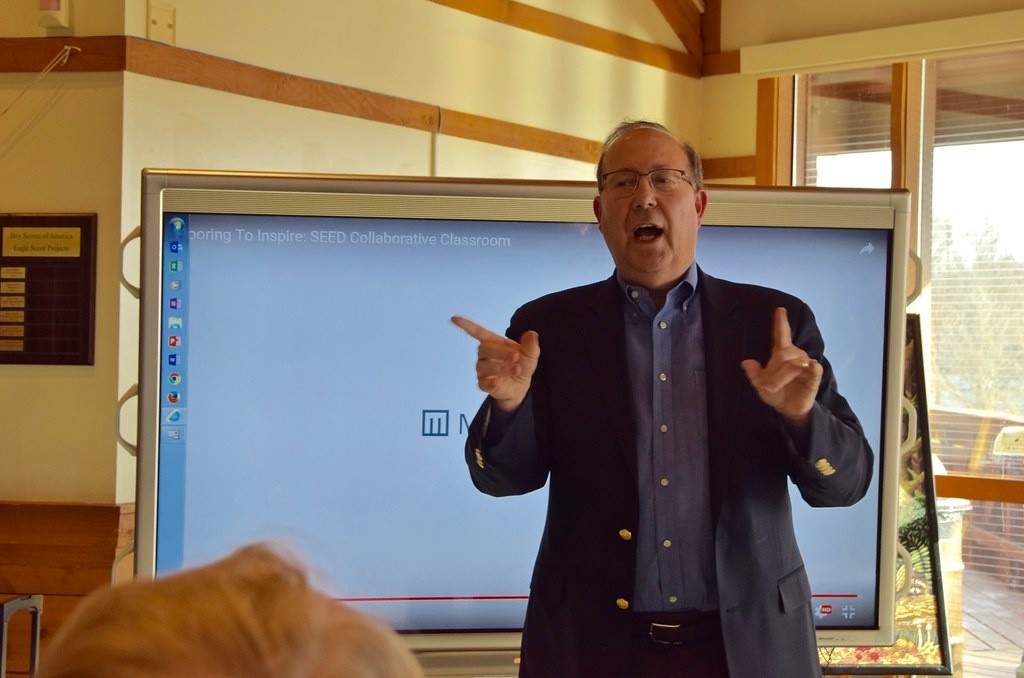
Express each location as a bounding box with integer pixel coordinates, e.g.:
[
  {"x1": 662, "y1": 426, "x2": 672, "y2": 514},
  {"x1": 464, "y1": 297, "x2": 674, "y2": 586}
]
[{"x1": 630, "y1": 623, "x2": 721, "y2": 645}]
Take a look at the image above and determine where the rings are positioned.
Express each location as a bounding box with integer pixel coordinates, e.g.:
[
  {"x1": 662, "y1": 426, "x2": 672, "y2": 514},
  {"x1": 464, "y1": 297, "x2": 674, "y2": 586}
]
[{"x1": 800, "y1": 355, "x2": 809, "y2": 369}]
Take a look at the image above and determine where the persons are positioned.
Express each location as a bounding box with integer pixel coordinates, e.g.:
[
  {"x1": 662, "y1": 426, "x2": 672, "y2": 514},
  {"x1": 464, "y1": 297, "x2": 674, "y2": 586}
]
[
  {"x1": 452, "y1": 119, "x2": 874, "y2": 678},
  {"x1": 37, "y1": 541, "x2": 426, "y2": 678}
]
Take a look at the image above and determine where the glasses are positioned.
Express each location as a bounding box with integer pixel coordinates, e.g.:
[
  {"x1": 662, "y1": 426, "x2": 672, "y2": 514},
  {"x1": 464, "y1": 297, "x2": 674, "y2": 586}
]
[{"x1": 600, "y1": 168, "x2": 697, "y2": 197}]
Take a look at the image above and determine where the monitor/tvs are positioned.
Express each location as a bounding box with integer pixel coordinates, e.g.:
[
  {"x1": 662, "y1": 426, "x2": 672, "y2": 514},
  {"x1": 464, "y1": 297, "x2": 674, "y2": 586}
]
[{"x1": 134, "y1": 167, "x2": 912, "y2": 647}]
